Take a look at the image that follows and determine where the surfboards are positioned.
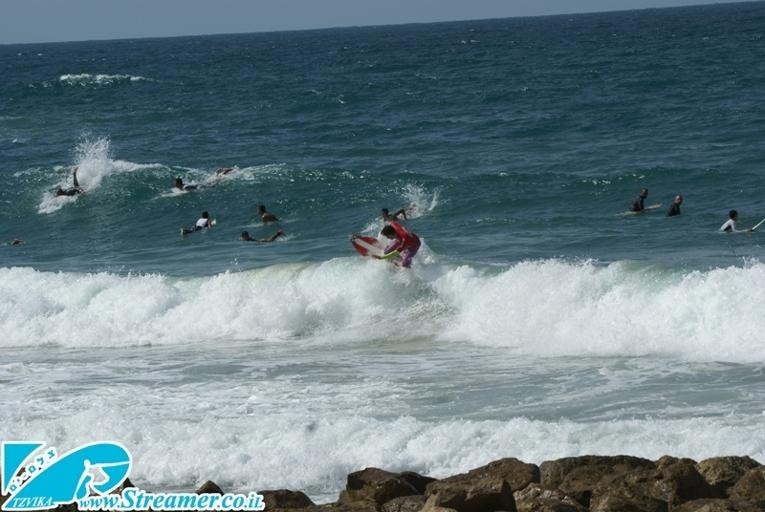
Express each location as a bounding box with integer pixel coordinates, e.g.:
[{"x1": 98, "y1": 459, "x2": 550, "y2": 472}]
[{"x1": 350, "y1": 233, "x2": 387, "y2": 258}]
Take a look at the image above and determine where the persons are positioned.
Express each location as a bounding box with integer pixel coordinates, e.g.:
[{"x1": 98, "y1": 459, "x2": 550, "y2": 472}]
[
  {"x1": 718, "y1": 209, "x2": 752, "y2": 233},
  {"x1": 174, "y1": 167, "x2": 232, "y2": 192},
  {"x1": 56, "y1": 166, "x2": 86, "y2": 196},
  {"x1": 256, "y1": 205, "x2": 279, "y2": 222},
  {"x1": 180, "y1": 211, "x2": 212, "y2": 234},
  {"x1": 371, "y1": 221, "x2": 421, "y2": 269},
  {"x1": 628, "y1": 187, "x2": 648, "y2": 212},
  {"x1": 667, "y1": 194, "x2": 683, "y2": 216},
  {"x1": 381, "y1": 206, "x2": 407, "y2": 221},
  {"x1": 240, "y1": 229, "x2": 283, "y2": 242}
]
